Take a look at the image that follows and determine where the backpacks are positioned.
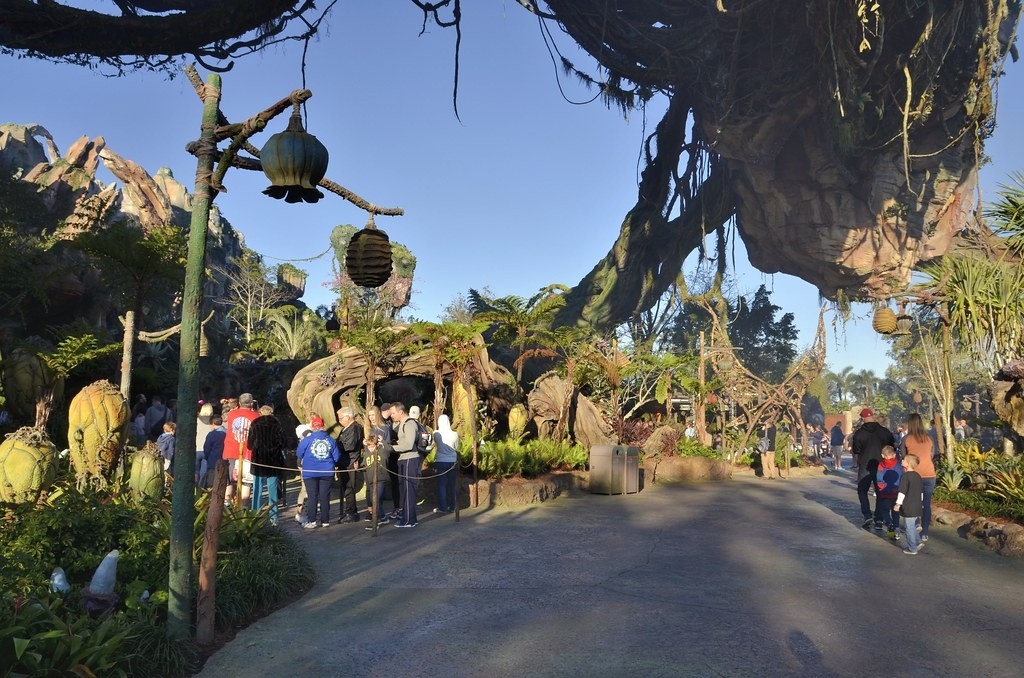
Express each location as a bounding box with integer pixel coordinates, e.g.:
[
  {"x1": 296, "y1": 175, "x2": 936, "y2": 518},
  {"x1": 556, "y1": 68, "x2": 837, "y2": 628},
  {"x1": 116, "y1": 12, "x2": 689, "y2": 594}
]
[
  {"x1": 758, "y1": 428, "x2": 770, "y2": 453},
  {"x1": 402, "y1": 418, "x2": 434, "y2": 452}
]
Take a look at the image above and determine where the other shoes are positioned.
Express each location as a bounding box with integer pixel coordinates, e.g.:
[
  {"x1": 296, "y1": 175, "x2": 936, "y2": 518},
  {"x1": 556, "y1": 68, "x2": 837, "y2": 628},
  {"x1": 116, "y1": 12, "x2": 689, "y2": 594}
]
[
  {"x1": 835, "y1": 467, "x2": 846, "y2": 470},
  {"x1": 760, "y1": 476, "x2": 775, "y2": 481},
  {"x1": 916, "y1": 543, "x2": 925, "y2": 551},
  {"x1": 433, "y1": 507, "x2": 447, "y2": 514},
  {"x1": 270, "y1": 517, "x2": 279, "y2": 528},
  {"x1": 337, "y1": 504, "x2": 419, "y2": 531},
  {"x1": 894, "y1": 528, "x2": 900, "y2": 540},
  {"x1": 447, "y1": 506, "x2": 454, "y2": 513},
  {"x1": 862, "y1": 517, "x2": 873, "y2": 528},
  {"x1": 921, "y1": 535, "x2": 929, "y2": 541},
  {"x1": 887, "y1": 522, "x2": 894, "y2": 537},
  {"x1": 915, "y1": 524, "x2": 923, "y2": 532},
  {"x1": 874, "y1": 521, "x2": 883, "y2": 530},
  {"x1": 304, "y1": 521, "x2": 317, "y2": 529},
  {"x1": 903, "y1": 547, "x2": 918, "y2": 555},
  {"x1": 296, "y1": 514, "x2": 309, "y2": 526},
  {"x1": 322, "y1": 523, "x2": 330, "y2": 527},
  {"x1": 851, "y1": 466, "x2": 860, "y2": 469}
]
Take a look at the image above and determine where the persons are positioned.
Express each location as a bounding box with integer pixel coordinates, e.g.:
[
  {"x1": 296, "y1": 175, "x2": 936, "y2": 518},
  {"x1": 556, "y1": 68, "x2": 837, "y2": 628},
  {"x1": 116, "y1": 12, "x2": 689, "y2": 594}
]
[
  {"x1": 685, "y1": 421, "x2": 695, "y2": 438},
  {"x1": 831, "y1": 421, "x2": 847, "y2": 470},
  {"x1": 806, "y1": 422, "x2": 829, "y2": 458},
  {"x1": 434, "y1": 414, "x2": 460, "y2": 513},
  {"x1": 853, "y1": 409, "x2": 897, "y2": 530},
  {"x1": 757, "y1": 416, "x2": 776, "y2": 479},
  {"x1": 893, "y1": 454, "x2": 925, "y2": 555},
  {"x1": 874, "y1": 445, "x2": 902, "y2": 540},
  {"x1": 778, "y1": 422, "x2": 789, "y2": 432},
  {"x1": 295, "y1": 401, "x2": 421, "y2": 529},
  {"x1": 848, "y1": 419, "x2": 973, "y2": 469},
  {"x1": 901, "y1": 413, "x2": 934, "y2": 541},
  {"x1": 388, "y1": 402, "x2": 421, "y2": 527},
  {"x1": 247, "y1": 405, "x2": 286, "y2": 526},
  {"x1": 130, "y1": 393, "x2": 258, "y2": 512}
]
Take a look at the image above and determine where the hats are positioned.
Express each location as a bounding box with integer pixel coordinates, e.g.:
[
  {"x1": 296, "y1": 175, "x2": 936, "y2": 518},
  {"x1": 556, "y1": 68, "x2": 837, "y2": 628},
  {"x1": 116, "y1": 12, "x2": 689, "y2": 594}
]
[
  {"x1": 859, "y1": 409, "x2": 873, "y2": 417},
  {"x1": 311, "y1": 417, "x2": 324, "y2": 428}
]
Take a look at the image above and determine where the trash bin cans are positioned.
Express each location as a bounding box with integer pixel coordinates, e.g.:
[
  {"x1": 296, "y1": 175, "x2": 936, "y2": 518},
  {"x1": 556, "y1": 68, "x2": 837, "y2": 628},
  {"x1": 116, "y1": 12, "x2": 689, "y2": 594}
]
[
  {"x1": 622, "y1": 446, "x2": 640, "y2": 495},
  {"x1": 589, "y1": 444, "x2": 624, "y2": 496}
]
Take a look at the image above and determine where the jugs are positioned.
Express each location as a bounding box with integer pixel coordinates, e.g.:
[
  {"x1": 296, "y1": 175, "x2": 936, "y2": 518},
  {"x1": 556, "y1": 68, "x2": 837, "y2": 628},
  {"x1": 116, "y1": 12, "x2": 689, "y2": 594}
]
[{"x1": 421, "y1": 432, "x2": 431, "y2": 446}]
